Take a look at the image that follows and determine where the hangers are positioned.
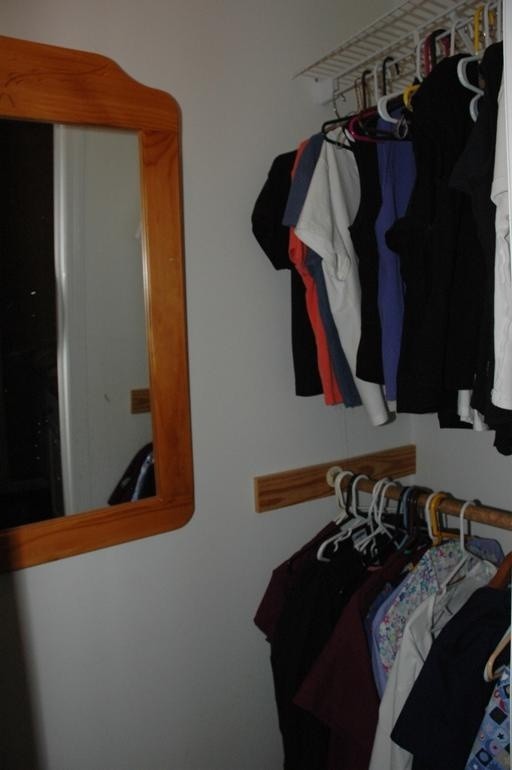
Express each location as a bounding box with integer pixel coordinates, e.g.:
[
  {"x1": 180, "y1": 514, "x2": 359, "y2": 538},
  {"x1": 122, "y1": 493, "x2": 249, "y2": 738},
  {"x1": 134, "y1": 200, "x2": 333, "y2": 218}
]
[
  {"x1": 317, "y1": 467, "x2": 512, "y2": 681},
  {"x1": 318, "y1": 6, "x2": 499, "y2": 149}
]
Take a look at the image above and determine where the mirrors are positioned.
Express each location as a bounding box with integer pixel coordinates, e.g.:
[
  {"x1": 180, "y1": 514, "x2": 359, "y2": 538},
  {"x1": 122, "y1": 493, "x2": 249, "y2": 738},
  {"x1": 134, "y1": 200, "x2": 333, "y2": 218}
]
[{"x1": 1, "y1": 34, "x2": 196, "y2": 573}]
